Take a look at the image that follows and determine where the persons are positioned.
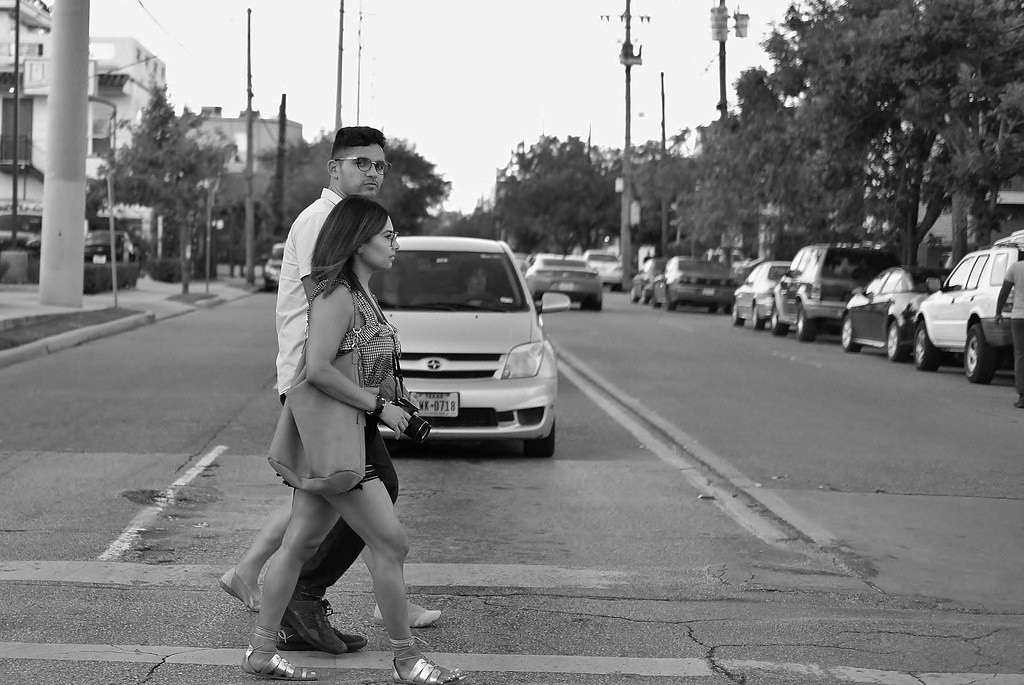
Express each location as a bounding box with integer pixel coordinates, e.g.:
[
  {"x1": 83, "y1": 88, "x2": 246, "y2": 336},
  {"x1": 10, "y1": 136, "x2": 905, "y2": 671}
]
[
  {"x1": 218, "y1": 492, "x2": 441, "y2": 630},
  {"x1": 994, "y1": 260, "x2": 1024, "y2": 408},
  {"x1": 276, "y1": 126, "x2": 395, "y2": 657},
  {"x1": 242, "y1": 193, "x2": 465, "y2": 685}
]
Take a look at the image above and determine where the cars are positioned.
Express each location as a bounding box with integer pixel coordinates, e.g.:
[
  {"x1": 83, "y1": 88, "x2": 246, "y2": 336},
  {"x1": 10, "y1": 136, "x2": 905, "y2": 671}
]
[
  {"x1": 0, "y1": 232, "x2": 40, "y2": 250},
  {"x1": 732, "y1": 260, "x2": 795, "y2": 330},
  {"x1": 369, "y1": 236, "x2": 559, "y2": 458},
  {"x1": 84, "y1": 233, "x2": 133, "y2": 263},
  {"x1": 651, "y1": 256, "x2": 735, "y2": 315},
  {"x1": 262, "y1": 242, "x2": 286, "y2": 289},
  {"x1": 838, "y1": 266, "x2": 950, "y2": 363},
  {"x1": 523, "y1": 253, "x2": 603, "y2": 313},
  {"x1": 631, "y1": 259, "x2": 668, "y2": 303}
]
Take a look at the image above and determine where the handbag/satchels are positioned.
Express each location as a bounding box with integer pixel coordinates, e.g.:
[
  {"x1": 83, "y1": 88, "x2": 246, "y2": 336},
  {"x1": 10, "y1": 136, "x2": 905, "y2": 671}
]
[{"x1": 267, "y1": 279, "x2": 366, "y2": 496}]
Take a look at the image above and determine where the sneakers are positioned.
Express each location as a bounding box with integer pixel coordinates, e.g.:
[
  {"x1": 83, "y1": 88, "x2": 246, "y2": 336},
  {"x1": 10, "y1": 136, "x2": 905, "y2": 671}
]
[
  {"x1": 276, "y1": 624, "x2": 367, "y2": 651},
  {"x1": 286, "y1": 585, "x2": 348, "y2": 654}
]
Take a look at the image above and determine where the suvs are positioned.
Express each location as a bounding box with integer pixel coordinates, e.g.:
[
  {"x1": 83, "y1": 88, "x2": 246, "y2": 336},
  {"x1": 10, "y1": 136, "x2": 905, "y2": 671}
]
[
  {"x1": 913, "y1": 246, "x2": 1024, "y2": 383},
  {"x1": 770, "y1": 243, "x2": 901, "y2": 342},
  {"x1": 583, "y1": 251, "x2": 624, "y2": 294}
]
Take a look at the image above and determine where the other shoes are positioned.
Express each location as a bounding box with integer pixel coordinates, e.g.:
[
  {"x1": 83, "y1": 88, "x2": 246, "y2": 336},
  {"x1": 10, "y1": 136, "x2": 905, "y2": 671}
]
[
  {"x1": 374, "y1": 601, "x2": 441, "y2": 627},
  {"x1": 219, "y1": 566, "x2": 261, "y2": 613}
]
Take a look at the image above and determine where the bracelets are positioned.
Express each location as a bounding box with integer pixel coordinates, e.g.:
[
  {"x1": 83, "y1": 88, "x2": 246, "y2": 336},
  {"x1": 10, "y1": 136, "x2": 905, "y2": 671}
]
[{"x1": 367, "y1": 395, "x2": 385, "y2": 417}]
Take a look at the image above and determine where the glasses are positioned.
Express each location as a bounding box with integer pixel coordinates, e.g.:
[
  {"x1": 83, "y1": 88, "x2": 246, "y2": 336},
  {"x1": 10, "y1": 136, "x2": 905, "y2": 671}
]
[
  {"x1": 335, "y1": 157, "x2": 392, "y2": 175},
  {"x1": 362, "y1": 232, "x2": 399, "y2": 246}
]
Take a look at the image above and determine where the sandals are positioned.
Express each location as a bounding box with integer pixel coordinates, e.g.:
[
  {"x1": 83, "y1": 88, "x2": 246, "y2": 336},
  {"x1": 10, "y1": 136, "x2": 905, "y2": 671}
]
[
  {"x1": 389, "y1": 636, "x2": 466, "y2": 685},
  {"x1": 243, "y1": 626, "x2": 318, "y2": 681}
]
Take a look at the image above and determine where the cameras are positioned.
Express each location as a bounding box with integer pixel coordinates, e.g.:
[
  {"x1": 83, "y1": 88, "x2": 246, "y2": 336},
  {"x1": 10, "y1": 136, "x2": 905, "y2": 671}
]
[{"x1": 383, "y1": 397, "x2": 432, "y2": 445}]
